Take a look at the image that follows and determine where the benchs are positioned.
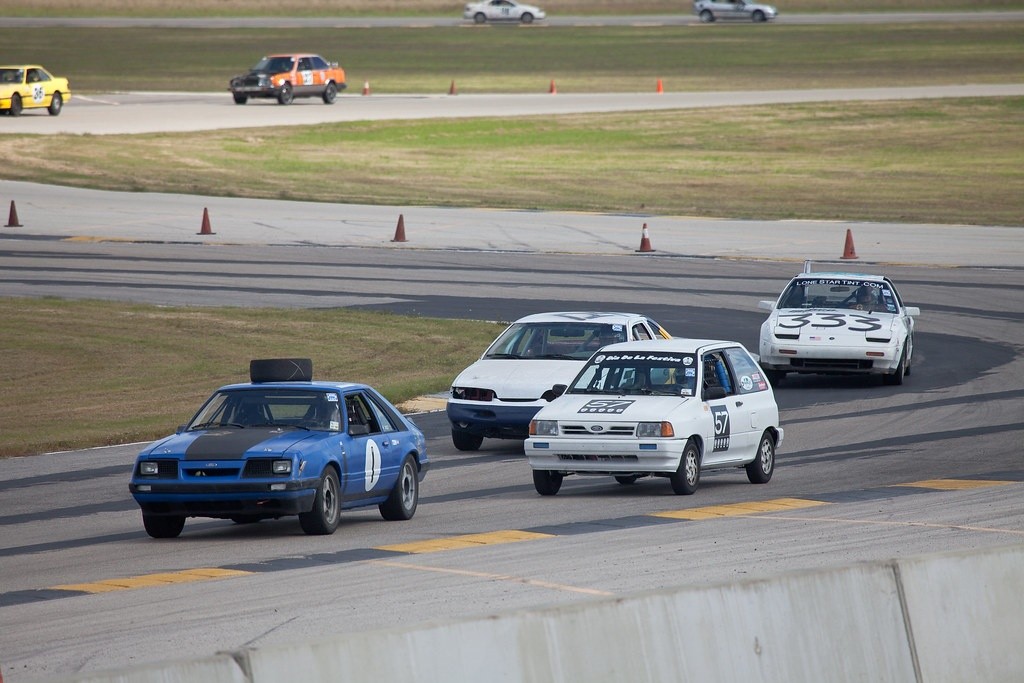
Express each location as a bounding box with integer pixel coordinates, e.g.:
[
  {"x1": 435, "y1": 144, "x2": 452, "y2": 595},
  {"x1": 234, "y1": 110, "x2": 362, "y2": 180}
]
[{"x1": 544, "y1": 340, "x2": 600, "y2": 354}]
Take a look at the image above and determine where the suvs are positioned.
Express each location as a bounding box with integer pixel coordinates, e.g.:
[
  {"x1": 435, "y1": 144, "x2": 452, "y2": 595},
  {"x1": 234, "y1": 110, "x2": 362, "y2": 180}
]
[{"x1": 694, "y1": 0, "x2": 778, "y2": 23}]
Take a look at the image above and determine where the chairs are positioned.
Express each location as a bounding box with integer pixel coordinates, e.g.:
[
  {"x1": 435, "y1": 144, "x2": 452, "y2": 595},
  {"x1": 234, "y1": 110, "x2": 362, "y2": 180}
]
[
  {"x1": 811, "y1": 295, "x2": 829, "y2": 307},
  {"x1": 232, "y1": 404, "x2": 272, "y2": 429}
]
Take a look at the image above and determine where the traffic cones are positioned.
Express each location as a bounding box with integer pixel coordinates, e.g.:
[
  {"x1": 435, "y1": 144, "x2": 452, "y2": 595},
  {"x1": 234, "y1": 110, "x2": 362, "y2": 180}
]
[
  {"x1": 196, "y1": 207, "x2": 217, "y2": 235},
  {"x1": 389, "y1": 214, "x2": 410, "y2": 243},
  {"x1": 3, "y1": 200, "x2": 24, "y2": 228},
  {"x1": 656, "y1": 80, "x2": 664, "y2": 94},
  {"x1": 449, "y1": 79, "x2": 458, "y2": 96},
  {"x1": 362, "y1": 81, "x2": 371, "y2": 96},
  {"x1": 839, "y1": 228, "x2": 859, "y2": 259},
  {"x1": 547, "y1": 80, "x2": 556, "y2": 95},
  {"x1": 635, "y1": 223, "x2": 656, "y2": 252}
]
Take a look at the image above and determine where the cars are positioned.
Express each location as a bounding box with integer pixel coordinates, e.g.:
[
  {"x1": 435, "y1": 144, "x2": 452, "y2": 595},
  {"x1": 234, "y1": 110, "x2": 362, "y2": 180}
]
[
  {"x1": 445, "y1": 310, "x2": 673, "y2": 452},
  {"x1": 0, "y1": 64, "x2": 72, "y2": 116},
  {"x1": 227, "y1": 54, "x2": 347, "y2": 106},
  {"x1": 129, "y1": 358, "x2": 430, "y2": 539},
  {"x1": 759, "y1": 260, "x2": 921, "y2": 388},
  {"x1": 525, "y1": 339, "x2": 784, "y2": 495},
  {"x1": 464, "y1": 0, "x2": 545, "y2": 25}
]
